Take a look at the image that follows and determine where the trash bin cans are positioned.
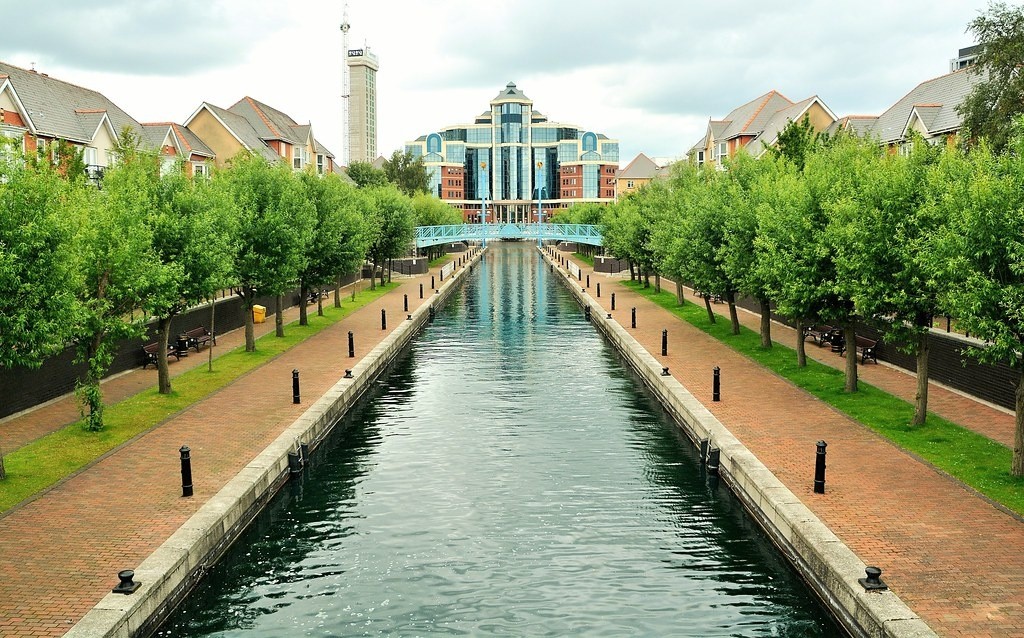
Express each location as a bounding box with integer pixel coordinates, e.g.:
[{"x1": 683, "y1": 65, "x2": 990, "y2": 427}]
[
  {"x1": 252, "y1": 304, "x2": 268, "y2": 324},
  {"x1": 830, "y1": 329, "x2": 843, "y2": 352},
  {"x1": 311, "y1": 293, "x2": 317, "y2": 304},
  {"x1": 176, "y1": 334, "x2": 189, "y2": 357}
]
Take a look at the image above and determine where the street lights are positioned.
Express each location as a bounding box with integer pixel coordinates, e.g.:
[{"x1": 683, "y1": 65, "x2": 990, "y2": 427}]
[
  {"x1": 532, "y1": 161, "x2": 548, "y2": 250},
  {"x1": 476, "y1": 162, "x2": 491, "y2": 248}
]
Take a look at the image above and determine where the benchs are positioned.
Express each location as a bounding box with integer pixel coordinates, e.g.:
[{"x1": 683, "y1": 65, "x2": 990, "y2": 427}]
[
  {"x1": 839, "y1": 332, "x2": 879, "y2": 366},
  {"x1": 183, "y1": 323, "x2": 217, "y2": 353},
  {"x1": 693, "y1": 290, "x2": 705, "y2": 298},
  {"x1": 139, "y1": 342, "x2": 180, "y2": 370},
  {"x1": 804, "y1": 324, "x2": 836, "y2": 348},
  {"x1": 308, "y1": 290, "x2": 329, "y2": 298},
  {"x1": 709, "y1": 294, "x2": 724, "y2": 304},
  {"x1": 297, "y1": 292, "x2": 316, "y2": 307}
]
